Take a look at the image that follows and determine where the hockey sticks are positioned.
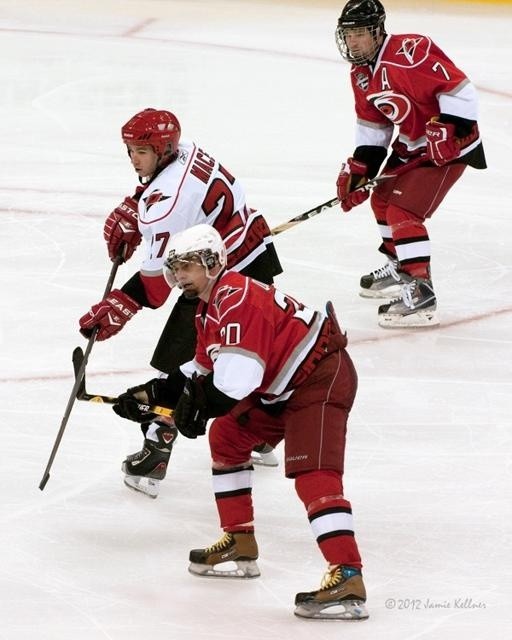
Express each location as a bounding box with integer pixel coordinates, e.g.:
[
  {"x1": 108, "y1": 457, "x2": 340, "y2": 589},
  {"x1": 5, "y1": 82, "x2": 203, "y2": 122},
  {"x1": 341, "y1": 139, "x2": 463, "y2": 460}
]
[{"x1": 74, "y1": 347, "x2": 173, "y2": 418}]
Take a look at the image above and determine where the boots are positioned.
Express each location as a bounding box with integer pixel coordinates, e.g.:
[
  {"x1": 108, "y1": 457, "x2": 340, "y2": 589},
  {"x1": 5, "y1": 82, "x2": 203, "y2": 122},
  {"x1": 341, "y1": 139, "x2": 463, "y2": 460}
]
[
  {"x1": 186, "y1": 528, "x2": 262, "y2": 580},
  {"x1": 359, "y1": 261, "x2": 413, "y2": 298},
  {"x1": 119, "y1": 419, "x2": 179, "y2": 499},
  {"x1": 293, "y1": 565, "x2": 370, "y2": 621},
  {"x1": 376, "y1": 279, "x2": 440, "y2": 328}
]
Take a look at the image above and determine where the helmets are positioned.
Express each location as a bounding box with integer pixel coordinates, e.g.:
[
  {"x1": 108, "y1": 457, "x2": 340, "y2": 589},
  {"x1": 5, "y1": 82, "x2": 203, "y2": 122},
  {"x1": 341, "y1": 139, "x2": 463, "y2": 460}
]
[
  {"x1": 334, "y1": 0, "x2": 386, "y2": 65},
  {"x1": 121, "y1": 107, "x2": 183, "y2": 156},
  {"x1": 161, "y1": 224, "x2": 229, "y2": 281}
]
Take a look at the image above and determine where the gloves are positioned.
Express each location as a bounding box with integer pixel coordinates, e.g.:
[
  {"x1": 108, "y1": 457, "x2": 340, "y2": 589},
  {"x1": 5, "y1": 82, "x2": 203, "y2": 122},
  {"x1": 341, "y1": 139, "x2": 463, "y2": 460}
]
[
  {"x1": 109, "y1": 379, "x2": 178, "y2": 424},
  {"x1": 173, "y1": 380, "x2": 219, "y2": 440},
  {"x1": 336, "y1": 157, "x2": 379, "y2": 215},
  {"x1": 80, "y1": 289, "x2": 144, "y2": 344},
  {"x1": 105, "y1": 200, "x2": 143, "y2": 265}
]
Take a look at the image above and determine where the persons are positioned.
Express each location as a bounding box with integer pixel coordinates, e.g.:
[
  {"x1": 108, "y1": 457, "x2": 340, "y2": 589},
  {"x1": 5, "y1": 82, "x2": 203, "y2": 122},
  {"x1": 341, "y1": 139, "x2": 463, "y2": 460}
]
[
  {"x1": 114, "y1": 221, "x2": 368, "y2": 607},
  {"x1": 335, "y1": 0, "x2": 487, "y2": 316},
  {"x1": 79, "y1": 108, "x2": 282, "y2": 482}
]
